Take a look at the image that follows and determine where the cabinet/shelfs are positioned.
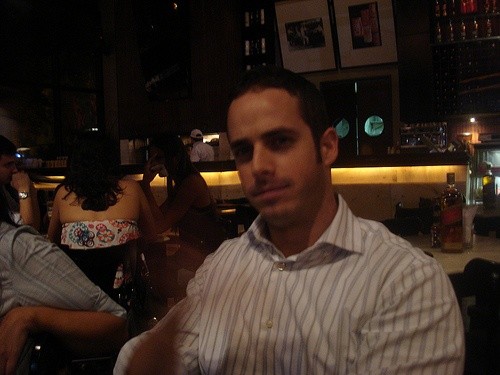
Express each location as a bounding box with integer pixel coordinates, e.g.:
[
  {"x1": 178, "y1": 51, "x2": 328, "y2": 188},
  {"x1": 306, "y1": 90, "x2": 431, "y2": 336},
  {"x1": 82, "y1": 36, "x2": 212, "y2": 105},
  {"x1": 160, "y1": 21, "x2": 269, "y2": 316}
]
[
  {"x1": 400, "y1": 130, "x2": 446, "y2": 149},
  {"x1": 428, "y1": 0, "x2": 500, "y2": 117},
  {"x1": 469, "y1": 141, "x2": 500, "y2": 205},
  {"x1": 240, "y1": 5, "x2": 274, "y2": 64}
]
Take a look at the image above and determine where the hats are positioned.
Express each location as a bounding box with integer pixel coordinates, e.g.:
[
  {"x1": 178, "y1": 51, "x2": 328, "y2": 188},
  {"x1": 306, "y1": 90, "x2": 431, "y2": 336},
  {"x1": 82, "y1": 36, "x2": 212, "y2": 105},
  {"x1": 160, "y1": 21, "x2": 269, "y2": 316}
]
[{"x1": 190, "y1": 129, "x2": 202, "y2": 138}]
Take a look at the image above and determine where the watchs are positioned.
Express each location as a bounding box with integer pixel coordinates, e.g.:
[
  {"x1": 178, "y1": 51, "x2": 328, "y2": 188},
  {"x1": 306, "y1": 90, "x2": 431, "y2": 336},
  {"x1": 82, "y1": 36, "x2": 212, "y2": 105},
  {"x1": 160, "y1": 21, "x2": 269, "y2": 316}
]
[{"x1": 19, "y1": 192, "x2": 32, "y2": 200}]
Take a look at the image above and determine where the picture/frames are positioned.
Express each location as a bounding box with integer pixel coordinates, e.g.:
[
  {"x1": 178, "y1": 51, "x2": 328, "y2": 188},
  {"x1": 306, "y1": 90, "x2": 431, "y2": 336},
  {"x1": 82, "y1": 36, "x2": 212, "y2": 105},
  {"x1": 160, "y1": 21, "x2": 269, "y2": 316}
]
[{"x1": 274, "y1": 0, "x2": 400, "y2": 75}]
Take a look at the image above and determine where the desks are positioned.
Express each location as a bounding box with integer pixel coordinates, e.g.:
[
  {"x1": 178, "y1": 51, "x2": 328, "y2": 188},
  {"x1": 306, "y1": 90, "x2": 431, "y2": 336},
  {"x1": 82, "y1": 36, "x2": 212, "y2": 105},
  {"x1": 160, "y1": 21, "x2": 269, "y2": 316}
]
[{"x1": 403, "y1": 233, "x2": 500, "y2": 275}]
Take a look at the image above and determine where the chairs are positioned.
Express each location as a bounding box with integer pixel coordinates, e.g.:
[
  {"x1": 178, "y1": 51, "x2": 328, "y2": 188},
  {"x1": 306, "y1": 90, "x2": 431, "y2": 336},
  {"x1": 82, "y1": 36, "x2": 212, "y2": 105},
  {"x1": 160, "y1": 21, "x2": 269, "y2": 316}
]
[
  {"x1": 55, "y1": 240, "x2": 139, "y2": 375},
  {"x1": 13, "y1": 327, "x2": 75, "y2": 375},
  {"x1": 231, "y1": 202, "x2": 258, "y2": 235},
  {"x1": 384, "y1": 202, "x2": 500, "y2": 375}
]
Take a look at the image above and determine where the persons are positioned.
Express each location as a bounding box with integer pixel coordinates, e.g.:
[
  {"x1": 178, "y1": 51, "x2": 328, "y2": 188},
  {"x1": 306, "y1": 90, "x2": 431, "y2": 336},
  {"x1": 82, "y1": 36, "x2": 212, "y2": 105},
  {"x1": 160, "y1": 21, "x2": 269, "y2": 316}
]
[
  {"x1": 47, "y1": 132, "x2": 153, "y2": 309},
  {"x1": 0, "y1": 134, "x2": 40, "y2": 232},
  {"x1": 0, "y1": 179, "x2": 127, "y2": 375},
  {"x1": 126, "y1": 65, "x2": 465, "y2": 375},
  {"x1": 188, "y1": 129, "x2": 214, "y2": 162},
  {"x1": 142, "y1": 133, "x2": 220, "y2": 271}
]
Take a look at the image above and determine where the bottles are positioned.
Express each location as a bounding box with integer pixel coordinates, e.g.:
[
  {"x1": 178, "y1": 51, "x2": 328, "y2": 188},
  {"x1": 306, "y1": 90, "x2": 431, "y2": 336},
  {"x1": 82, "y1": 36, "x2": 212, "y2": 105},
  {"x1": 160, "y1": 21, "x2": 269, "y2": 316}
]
[
  {"x1": 433, "y1": 0, "x2": 500, "y2": 44},
  {"x1": 438, "y1": 172, "x2": 464, "y2": 254},
  {"x1": 483, "y1": 164, "x2": 496, "y2": 206}
]
